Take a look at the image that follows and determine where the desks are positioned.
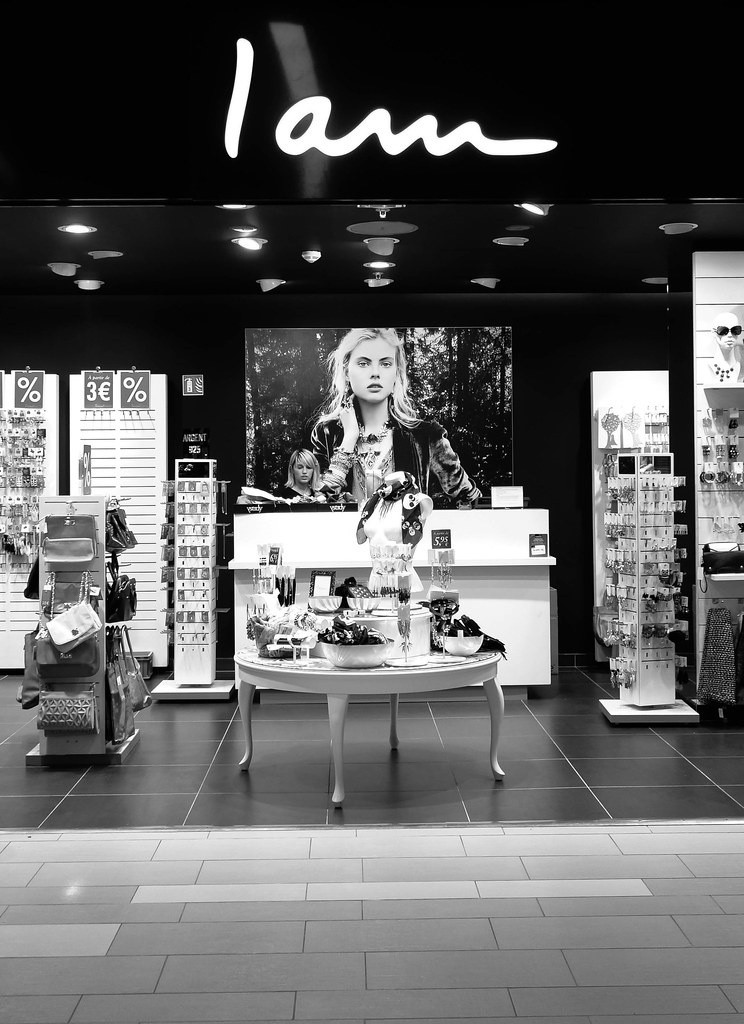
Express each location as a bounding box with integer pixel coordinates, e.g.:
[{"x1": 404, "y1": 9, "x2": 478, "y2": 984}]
[
  {"x1": 233, "y1": 643, "x2": 504, "y2": 807},
  {"x1": 227, "y1": 507, "x2": 560, "y2": 705}
]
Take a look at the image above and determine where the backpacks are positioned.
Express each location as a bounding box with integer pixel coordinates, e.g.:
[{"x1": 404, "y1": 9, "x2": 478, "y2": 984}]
[{"x1": 101, "y1": 556, "x2": 136, "y2": 623}]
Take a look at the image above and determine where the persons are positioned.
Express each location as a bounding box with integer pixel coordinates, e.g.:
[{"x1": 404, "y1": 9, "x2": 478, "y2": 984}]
[
  {"x1": 701, "y1": 312, "x2": 744, "y2": 383},
  {"x1": 271, "y1": 448, "x2": 321, "y2": 503},
  {"x1": 302, "y1": 328, "x2": 484, "y2": 508},
  {"x1": 355, "y1": 470, "x2": 434, "y2": 592}
]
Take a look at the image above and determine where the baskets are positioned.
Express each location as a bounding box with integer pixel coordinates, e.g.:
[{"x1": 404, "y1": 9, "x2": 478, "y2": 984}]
[{"x1": 250, "y1": 616, "x2": 301, "y2": 659}]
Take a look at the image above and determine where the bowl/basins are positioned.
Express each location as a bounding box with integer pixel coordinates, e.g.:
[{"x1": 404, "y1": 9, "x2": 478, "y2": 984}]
[
  {"x1": 439, "y1": 630, "x2": 485, "y2": 657},
  {"x1": 308, "y1": 595, "x2": 342, "y2": 614},
  {"x1": 321, "y1": 638, "x2": 397, "y2": 669},
  {"x1": 347, "y1": 596, "x2": 381, "y2": 614}
]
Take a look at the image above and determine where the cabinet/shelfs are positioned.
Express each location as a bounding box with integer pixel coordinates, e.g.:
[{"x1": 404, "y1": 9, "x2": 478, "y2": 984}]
[{"x1": 703, "y1": 380, "x2": 744, "y2": 586}]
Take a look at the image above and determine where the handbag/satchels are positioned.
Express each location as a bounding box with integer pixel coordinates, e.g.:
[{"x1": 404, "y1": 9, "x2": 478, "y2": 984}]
[
  {"x1": 35, "y1": 572, "x2": 101, "y2": 679},
  {"x1": 102, "y1": 506, "x2": 140, "y2": 554},
  {"x1": 47, "y1": 572, "x2": 103, "y2": 653},
  {"x1": 23, "y1": 560, "x2": 40, "y2": 600},
  {"x1": 36, "y1": 683, "x2": 95, "y2": 731},
  {"x1": 25, "y1": 626, "x2": 46, "y2": 711},
  {"x1": 106, "y1": 628, "x2": 153, "y2": 746},
  {"x1": 703, "y1": 543, "x2": 744, "y2": 574}
]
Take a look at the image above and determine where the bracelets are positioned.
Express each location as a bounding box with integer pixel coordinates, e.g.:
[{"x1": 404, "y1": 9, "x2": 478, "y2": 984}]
[{"x1": 323, "y1": 446, "x2": 353, "y2": 493}]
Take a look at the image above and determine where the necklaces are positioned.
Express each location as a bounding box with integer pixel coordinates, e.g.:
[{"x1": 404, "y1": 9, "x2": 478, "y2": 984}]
[{"x1": 359, "y1": 445, "x2": 382, "y2": 468}]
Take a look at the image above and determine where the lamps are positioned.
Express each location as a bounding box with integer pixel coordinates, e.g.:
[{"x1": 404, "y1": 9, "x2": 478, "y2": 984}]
[
  {"x1": 229, "y1": 236, "x2": 268, "y2": 249},
  {"x1": 659, "y1": 223, "x2": 697, "y2": 234},
  {"x1": 363, "y1": 237, "x2": 400, "y2": 255},
  {"x1": 256, "y1": 279, "x2": 286, "y2": 293},
  {"x1": 74, "y1": 279, "x2": 104, "y2": 290},
  {"x1": 86, "y1": 250, "x2": 122, "y2": 259},
  {"x1": 470, "y1": 277, "x2": 499, "y2": 288},
  {"x1": 47, "y1": 262, "x2": 81, "y2": 275},
  {"x1": 364, "y1": 279, "x2": 396, "y2": 288},
  {"x1": 512, "y1": 203, "x2": 553, "y2": 215},
  {"x1": 492, "y1": 236, "x2": 529, "y2": 246}
]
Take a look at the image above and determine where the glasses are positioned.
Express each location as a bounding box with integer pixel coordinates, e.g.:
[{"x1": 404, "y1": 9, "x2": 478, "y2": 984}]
[{"x1": 713, "y1": 326, "x2": 742, "y2": 335}]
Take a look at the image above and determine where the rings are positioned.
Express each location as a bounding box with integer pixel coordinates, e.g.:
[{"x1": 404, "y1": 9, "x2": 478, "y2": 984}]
[
  {"x1": 341, "y1": 401, "x2": 345, "y2": 409},
  {"x1": 347, "y1": 402, "x2": 354, "y2": 407},
  {"x1": 358, "y1": 421, "x2": 393, "y2": 444}
]
